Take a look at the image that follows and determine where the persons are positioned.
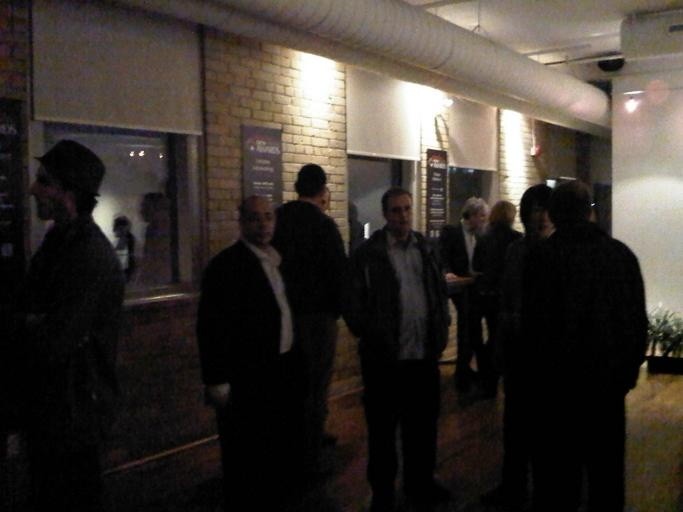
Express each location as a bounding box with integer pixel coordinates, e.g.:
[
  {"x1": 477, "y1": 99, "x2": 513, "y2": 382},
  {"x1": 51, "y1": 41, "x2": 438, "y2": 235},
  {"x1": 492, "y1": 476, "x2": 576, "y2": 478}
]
[
  {"x1": 139, "y1": 192, "x2": 171, "y2": 281},
  {"x1": 0, "y1": 138, "x2": 127, "y2": 511},
  {"x1": 108, "y1": 216, "x2": 135, "y2": 281}
]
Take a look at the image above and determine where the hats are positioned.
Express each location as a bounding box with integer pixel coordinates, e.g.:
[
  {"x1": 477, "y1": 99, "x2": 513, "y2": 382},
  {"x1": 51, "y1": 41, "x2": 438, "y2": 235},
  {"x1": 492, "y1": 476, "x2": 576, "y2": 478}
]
[{"x1": 33, "y1": 139, "x2": 106, "y2": 197}]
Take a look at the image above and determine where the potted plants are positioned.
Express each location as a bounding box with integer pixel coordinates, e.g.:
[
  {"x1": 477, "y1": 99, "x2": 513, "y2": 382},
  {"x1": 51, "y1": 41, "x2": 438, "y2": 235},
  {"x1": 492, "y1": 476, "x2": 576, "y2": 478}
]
[{"x1": 644, "y1": 312, "x2": 683, "y2": 374}]
